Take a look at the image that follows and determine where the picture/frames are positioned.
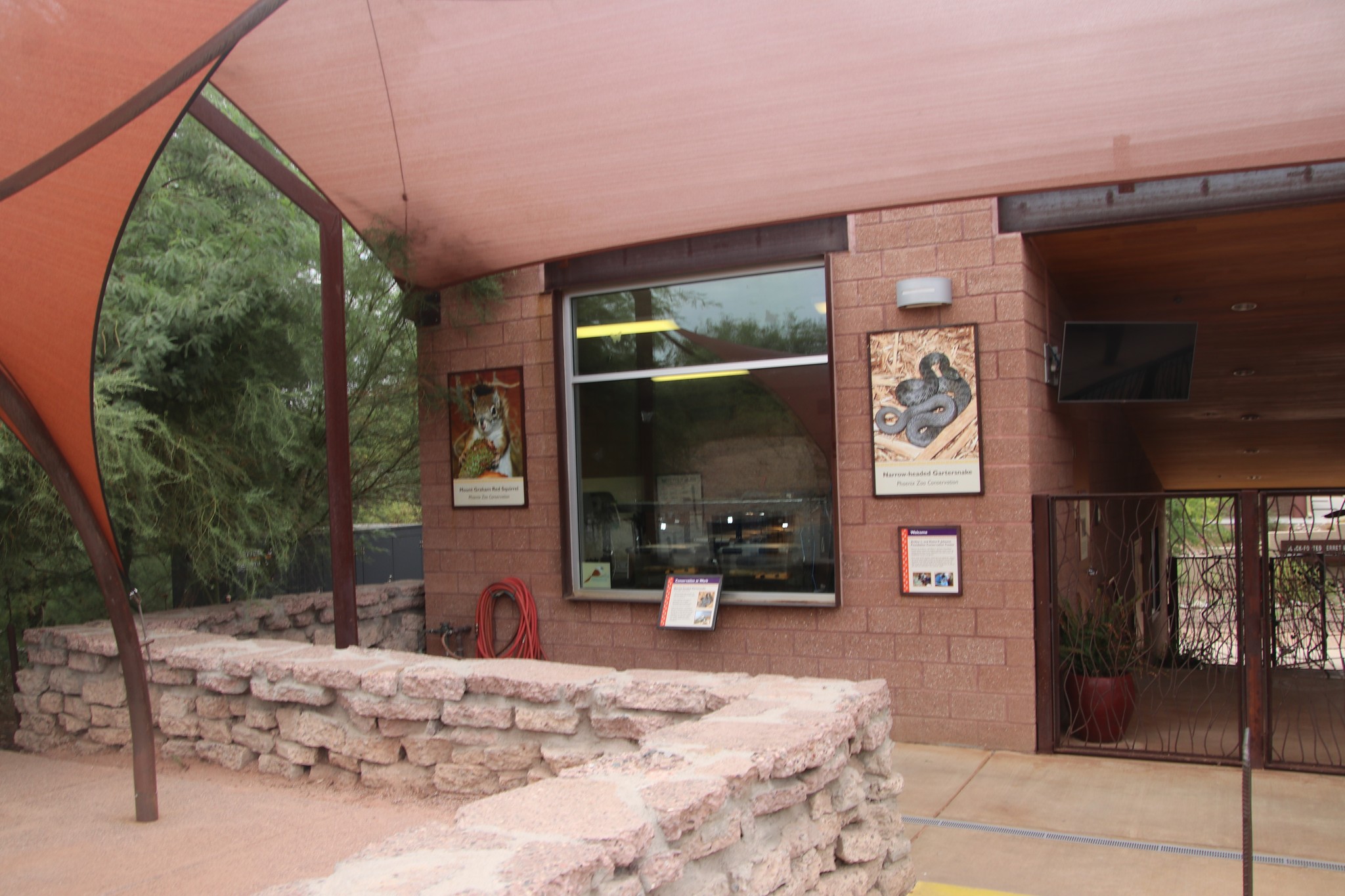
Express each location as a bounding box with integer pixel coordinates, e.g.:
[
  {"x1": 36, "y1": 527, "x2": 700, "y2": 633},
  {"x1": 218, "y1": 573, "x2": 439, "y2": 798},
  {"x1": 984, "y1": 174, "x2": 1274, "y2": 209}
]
[
  {"x1": 656, "y1": 570, "x2": 724, "y2": 632},
  {"x1": 447, "y1": 364, "x2": 530, "y2": 509},
  {"x1": 863, "y1": 322, "x2": 985, "y2": 497},
  {"x1": 897, "y1": 525, "x2": 963, "y2": 598}
]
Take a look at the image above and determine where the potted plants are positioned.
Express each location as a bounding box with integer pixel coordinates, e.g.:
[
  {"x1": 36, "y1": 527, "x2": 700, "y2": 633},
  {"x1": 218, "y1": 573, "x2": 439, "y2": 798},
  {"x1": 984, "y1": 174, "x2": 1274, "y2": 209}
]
[{"x1": 1055, "y1": 585, "x2": 1167, "y2": 742}]
